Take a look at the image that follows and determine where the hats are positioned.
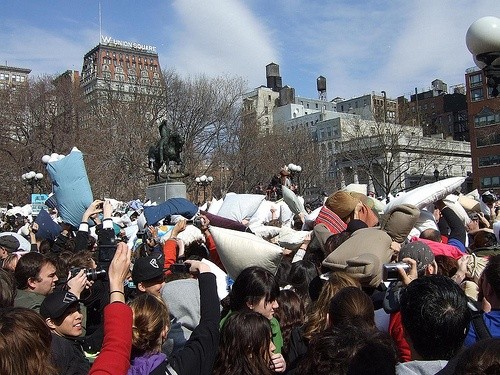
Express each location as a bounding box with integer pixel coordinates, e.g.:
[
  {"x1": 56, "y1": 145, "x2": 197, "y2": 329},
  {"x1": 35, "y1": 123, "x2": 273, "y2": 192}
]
[
  {"x1": 397, "y1": 241, "x2": 435, "y2": 272},
  {"x1": 132, "y1": 257, "x2": 169, "y2": 285},
  {"x1": 40, "y1": 291, "x2": 88, "y2": 320},
  {"x1": 325, "y1": 189, "x2": 360, "y2": 219},
  {"x1": 0, "y1": 234, "x2": 20, "y2": 252}
]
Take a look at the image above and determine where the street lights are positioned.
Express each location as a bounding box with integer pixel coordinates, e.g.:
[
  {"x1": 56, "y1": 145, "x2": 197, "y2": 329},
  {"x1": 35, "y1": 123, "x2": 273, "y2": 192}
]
[
  {"x1": 287, "y1": 163, "x2": 302, "y2": 194},
  {"x1": 194, "y1": 176, "x2": 213, "y2": 203},
  {"x1": 22, "y1": 171, "x2": 44, "y2": 193}
]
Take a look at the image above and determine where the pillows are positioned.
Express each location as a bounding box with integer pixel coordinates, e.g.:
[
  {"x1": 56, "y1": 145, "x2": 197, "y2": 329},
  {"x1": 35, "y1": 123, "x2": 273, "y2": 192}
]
[{"x1": 1, "y1": 146, "x2": 500, "y2": 329}]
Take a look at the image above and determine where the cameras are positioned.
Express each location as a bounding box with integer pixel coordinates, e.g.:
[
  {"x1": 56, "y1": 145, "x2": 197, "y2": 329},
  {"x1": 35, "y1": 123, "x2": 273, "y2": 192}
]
[
  {"x1": 136, "y1": 228, "x2": 152, "y2": 242},
  {"x1": 169, "y1": 263, "x2": 191, "y2": 273},
  {"x1": 99, "y1": 203, "x2": 103, "y2": 208},
  {"x1": 99, "y1": 245, "x2": 118, "y2": 262},
  {"x1": 186, "y1": 218, "x2": 202, "y2": 228},
  {"x1": 71, "y1": 265, "x2": 109, "y2": 286},
  {"x1": 382, "y1": 262, "x2": 412, "y2": 281}
]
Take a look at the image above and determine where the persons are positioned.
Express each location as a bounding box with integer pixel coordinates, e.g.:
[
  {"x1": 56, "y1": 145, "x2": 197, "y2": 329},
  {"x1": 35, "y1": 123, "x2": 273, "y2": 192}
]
[{"x1": 0, "y1": 190, "x2": 500, "y2": 375}]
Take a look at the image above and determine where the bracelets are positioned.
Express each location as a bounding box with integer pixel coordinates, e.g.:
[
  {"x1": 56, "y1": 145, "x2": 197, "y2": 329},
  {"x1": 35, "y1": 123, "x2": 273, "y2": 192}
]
[{"x1": 109, "y1": 290, "x2": 125, "y2": 296}]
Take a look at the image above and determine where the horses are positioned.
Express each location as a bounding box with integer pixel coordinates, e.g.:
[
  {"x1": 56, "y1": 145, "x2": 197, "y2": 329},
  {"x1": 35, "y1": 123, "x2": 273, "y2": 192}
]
[{"x1": 148, "y1": 131, "x2": 185, "y2": 182}]
